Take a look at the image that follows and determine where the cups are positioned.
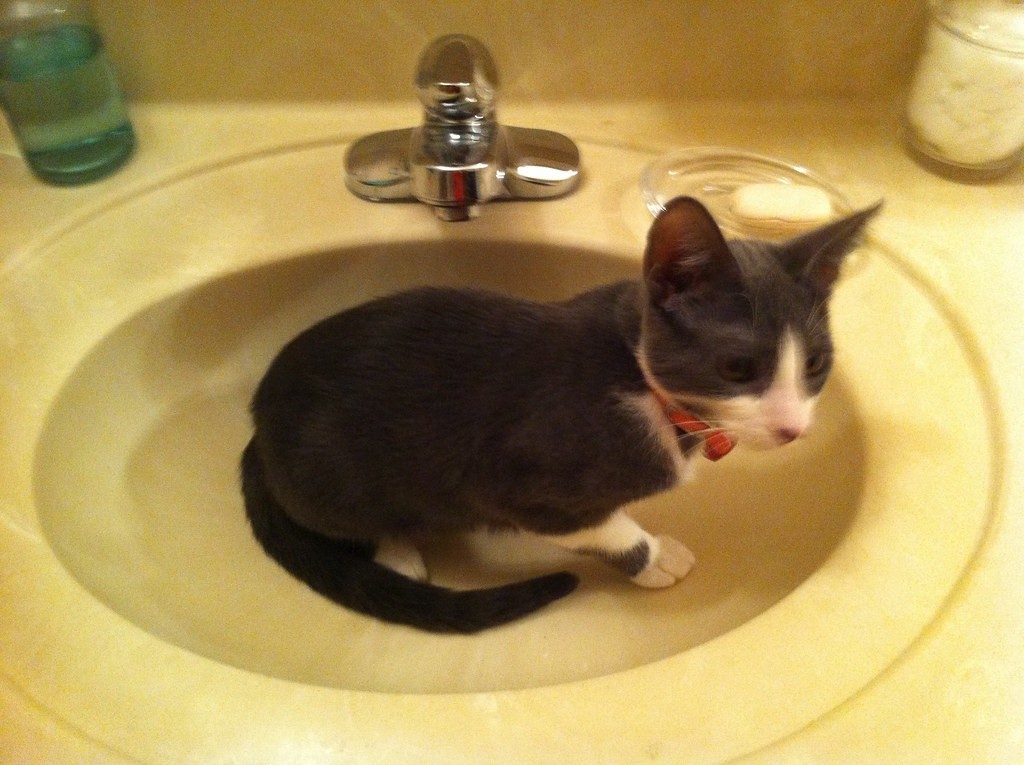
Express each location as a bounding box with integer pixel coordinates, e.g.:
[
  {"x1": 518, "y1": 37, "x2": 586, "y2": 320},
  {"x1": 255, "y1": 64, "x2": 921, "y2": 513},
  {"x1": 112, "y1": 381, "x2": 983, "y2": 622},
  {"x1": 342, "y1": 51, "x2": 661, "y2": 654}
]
[
  {"x1": 898, "y1": 1, "x2": 1024, "y2": 186},
  {"x1": 1, "y1": 1, "x2": 138, "y2": 189}
]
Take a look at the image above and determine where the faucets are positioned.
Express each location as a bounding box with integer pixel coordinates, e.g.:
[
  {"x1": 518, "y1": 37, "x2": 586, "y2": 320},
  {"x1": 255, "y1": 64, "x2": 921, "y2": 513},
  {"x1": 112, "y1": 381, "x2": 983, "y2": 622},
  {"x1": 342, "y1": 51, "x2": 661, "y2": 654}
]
[{"x1": 343, "y1": 33, "x2": 583, "y2": 223}]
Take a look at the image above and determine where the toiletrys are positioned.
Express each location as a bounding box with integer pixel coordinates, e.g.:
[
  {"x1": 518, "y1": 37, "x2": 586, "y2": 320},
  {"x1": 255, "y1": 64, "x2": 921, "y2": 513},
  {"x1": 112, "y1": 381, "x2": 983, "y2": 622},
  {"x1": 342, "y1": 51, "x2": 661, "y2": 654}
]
[{"x1": 1, "y1": 2, "x2": 137, "y2": 189}]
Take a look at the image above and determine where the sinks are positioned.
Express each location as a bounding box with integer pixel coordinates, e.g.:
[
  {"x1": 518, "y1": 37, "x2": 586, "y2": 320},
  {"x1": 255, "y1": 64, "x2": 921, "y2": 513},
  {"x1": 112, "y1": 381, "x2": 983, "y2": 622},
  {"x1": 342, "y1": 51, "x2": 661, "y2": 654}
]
[{"x1": 4, "y1": 123, "x2": 1004, "y2": 700}]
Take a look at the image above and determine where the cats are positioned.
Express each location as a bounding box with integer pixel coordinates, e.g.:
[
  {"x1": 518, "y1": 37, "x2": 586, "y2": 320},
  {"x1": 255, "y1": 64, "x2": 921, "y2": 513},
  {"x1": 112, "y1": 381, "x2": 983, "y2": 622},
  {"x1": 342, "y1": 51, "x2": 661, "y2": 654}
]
[{"x1": 235, "y1": 190, "x2": 889, "y2": 635}]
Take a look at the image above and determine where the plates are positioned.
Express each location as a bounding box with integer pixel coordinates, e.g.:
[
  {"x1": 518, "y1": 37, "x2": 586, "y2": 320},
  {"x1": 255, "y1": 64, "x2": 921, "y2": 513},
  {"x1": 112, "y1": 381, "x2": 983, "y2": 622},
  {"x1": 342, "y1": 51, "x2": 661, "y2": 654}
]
[{"x1": 639, "y1": 146, "x2": 870, "y2": 282}]
